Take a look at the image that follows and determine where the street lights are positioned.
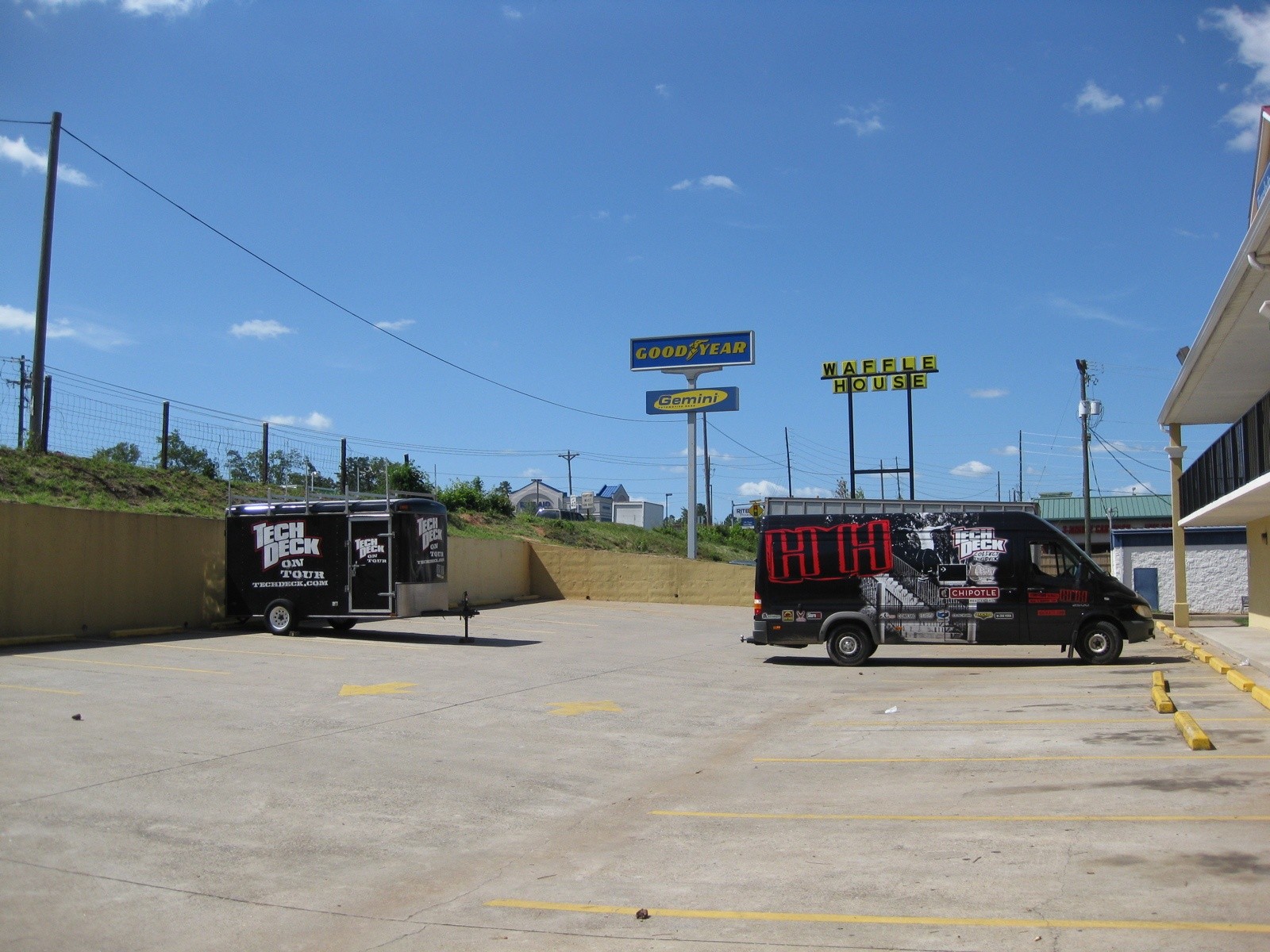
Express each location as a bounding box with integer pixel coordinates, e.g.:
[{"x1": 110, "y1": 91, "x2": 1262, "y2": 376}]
[
  {"x1": 531, "y1": 479, "x2": 542, "y2": 510},
  {"x1": 666, "y1": 493, "x2": 673, "y2": 518},
  {"x1": 1077, "y1": 398, "x2": 1102, "y2": 557}
]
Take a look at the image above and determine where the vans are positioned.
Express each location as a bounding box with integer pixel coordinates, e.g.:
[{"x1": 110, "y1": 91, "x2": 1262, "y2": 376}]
[
  {"x1": 740, "y1": 509, "x2": 1158, "y2": 669},
  {"x1": 535, "y1": 509, "x2": 585, "y2": 522}
]
